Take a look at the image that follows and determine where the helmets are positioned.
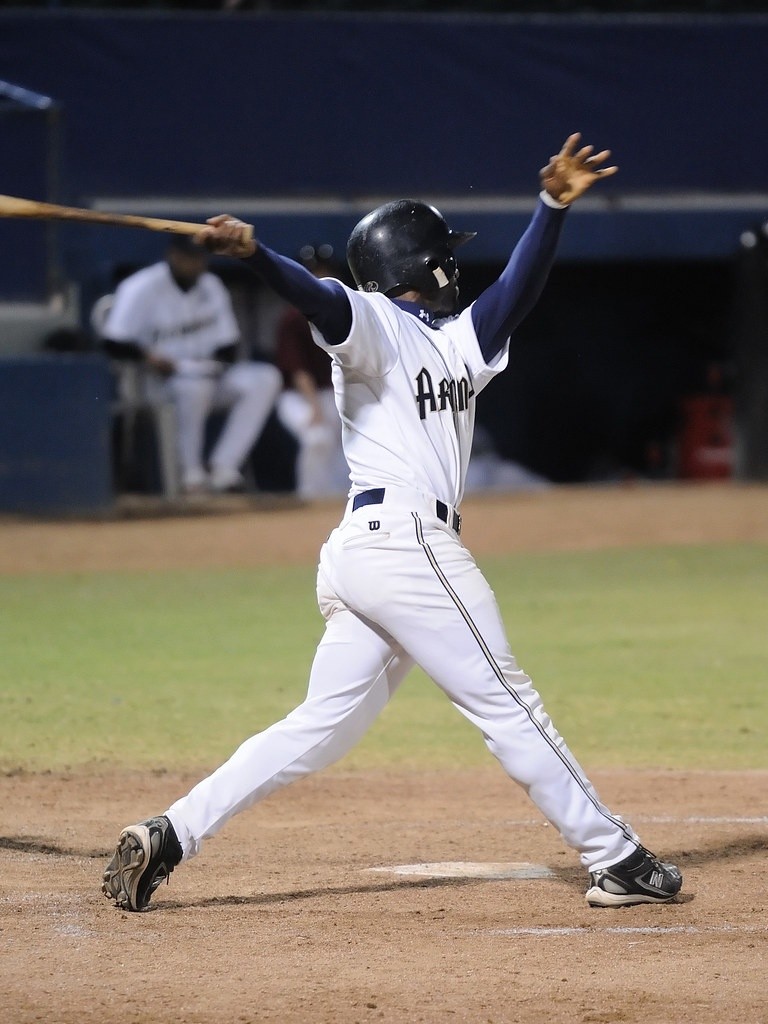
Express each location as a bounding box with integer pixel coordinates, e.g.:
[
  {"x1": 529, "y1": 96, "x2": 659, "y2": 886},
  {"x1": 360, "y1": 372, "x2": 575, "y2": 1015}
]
[{"x1": 346, "y1": 197, "x2": 477, "y2": 293}]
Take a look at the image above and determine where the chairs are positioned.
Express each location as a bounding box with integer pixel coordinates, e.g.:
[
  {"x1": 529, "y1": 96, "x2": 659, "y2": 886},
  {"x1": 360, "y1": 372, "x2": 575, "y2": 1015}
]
[{"x1": 68, "y1": 280, "x2": 258, "y2": 500}]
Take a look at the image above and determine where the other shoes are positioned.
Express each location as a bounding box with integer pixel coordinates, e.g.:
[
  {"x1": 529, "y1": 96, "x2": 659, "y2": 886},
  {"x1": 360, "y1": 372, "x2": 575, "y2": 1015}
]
[
  {"x1": 212, "y1": 469, "x2": 244, "y2": 491},
  {"x1": 181, "y1": 470, "x2": 211, "y2": 491}
]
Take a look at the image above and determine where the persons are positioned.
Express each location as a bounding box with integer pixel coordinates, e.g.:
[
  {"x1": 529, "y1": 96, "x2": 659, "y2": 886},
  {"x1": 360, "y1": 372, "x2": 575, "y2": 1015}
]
[
  {"x1": 103, "y1": 223, "x2": 283, "y2": 498},
  {"x1": 271, "y1": 238, "x2": 354, "y2": 498},
  {"x1": 100, "y1": 133, "x2": 682, "y2": 914}
]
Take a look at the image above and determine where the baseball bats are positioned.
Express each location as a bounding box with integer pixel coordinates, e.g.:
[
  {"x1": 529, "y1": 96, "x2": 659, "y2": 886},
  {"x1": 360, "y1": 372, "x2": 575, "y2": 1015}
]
[{"x1": 0, "y1": 178, "x2": 257, "y2": 253}]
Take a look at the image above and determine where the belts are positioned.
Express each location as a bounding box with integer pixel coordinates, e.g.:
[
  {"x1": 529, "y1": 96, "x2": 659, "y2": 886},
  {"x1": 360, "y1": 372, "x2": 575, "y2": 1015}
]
[{"x1": 352, "y1": 488, "x2": 462, "y2": 535}]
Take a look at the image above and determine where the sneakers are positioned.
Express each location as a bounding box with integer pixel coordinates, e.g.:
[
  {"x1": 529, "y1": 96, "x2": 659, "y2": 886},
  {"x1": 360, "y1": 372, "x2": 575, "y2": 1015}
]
[
  {"x1": 101, "y1": 815, "x2": 183, "y2": 913},
  {"x1": 585, "y1": 843, "x2": 683, "y2": 907}
]
